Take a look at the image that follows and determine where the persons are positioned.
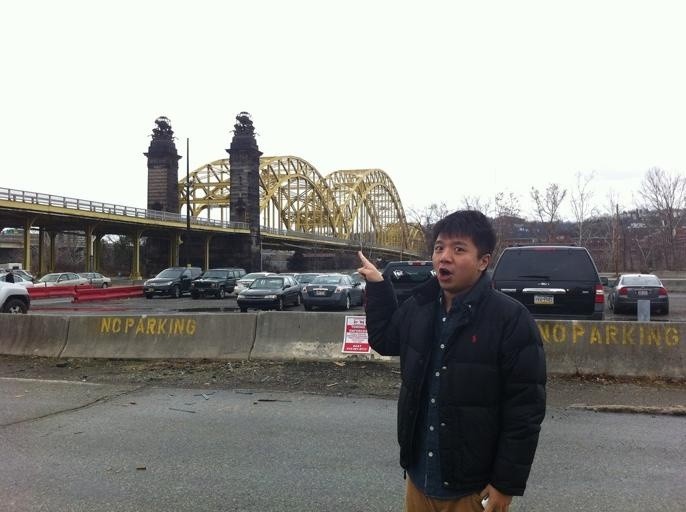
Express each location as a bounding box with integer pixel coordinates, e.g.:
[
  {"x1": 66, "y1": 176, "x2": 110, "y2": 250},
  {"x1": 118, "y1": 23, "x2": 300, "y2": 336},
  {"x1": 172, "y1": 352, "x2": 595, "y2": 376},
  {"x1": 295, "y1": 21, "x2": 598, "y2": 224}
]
[{"x1": 356, "y1": 208, "x2": 548, "y2": 511}]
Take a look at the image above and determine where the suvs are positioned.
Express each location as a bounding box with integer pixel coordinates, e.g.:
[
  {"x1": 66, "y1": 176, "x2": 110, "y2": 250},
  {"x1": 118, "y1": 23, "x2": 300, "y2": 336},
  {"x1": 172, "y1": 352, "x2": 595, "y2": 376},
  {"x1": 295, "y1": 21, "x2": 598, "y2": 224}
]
[
  {"x1": 142, "y1": 266, "x2": 202, "y2": 298},
  {"x1": 383, "y1": 261, "x2": 436, "y2": 304},
  {"x1": 191, "y1": 269, "x2": 247, "y2": 299}
]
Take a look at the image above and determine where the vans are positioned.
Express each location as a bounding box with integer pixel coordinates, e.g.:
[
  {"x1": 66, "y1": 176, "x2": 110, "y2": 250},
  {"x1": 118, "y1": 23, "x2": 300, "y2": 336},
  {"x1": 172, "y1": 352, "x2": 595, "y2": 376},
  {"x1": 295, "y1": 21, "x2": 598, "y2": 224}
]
[{"x1": 491, "y1": 245, "x2": 608, "y2": 320}]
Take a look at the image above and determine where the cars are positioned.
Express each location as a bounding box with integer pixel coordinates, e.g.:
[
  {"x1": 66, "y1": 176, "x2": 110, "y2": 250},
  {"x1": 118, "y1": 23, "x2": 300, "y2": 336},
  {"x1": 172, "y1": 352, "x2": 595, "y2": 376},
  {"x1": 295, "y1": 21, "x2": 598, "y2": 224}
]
[
  {"x1": 34, "y1": 272, "x2": 112, "y2": 288},
  {"x1": 607, "y1": 273, "x2": 669, "y2": 314},
  {"x1": 0, "y1": 268, "x2": 36, "y2": 313},
  {"x1": 233, "y1": 272, "x2": 366, "y2": 312}
]
